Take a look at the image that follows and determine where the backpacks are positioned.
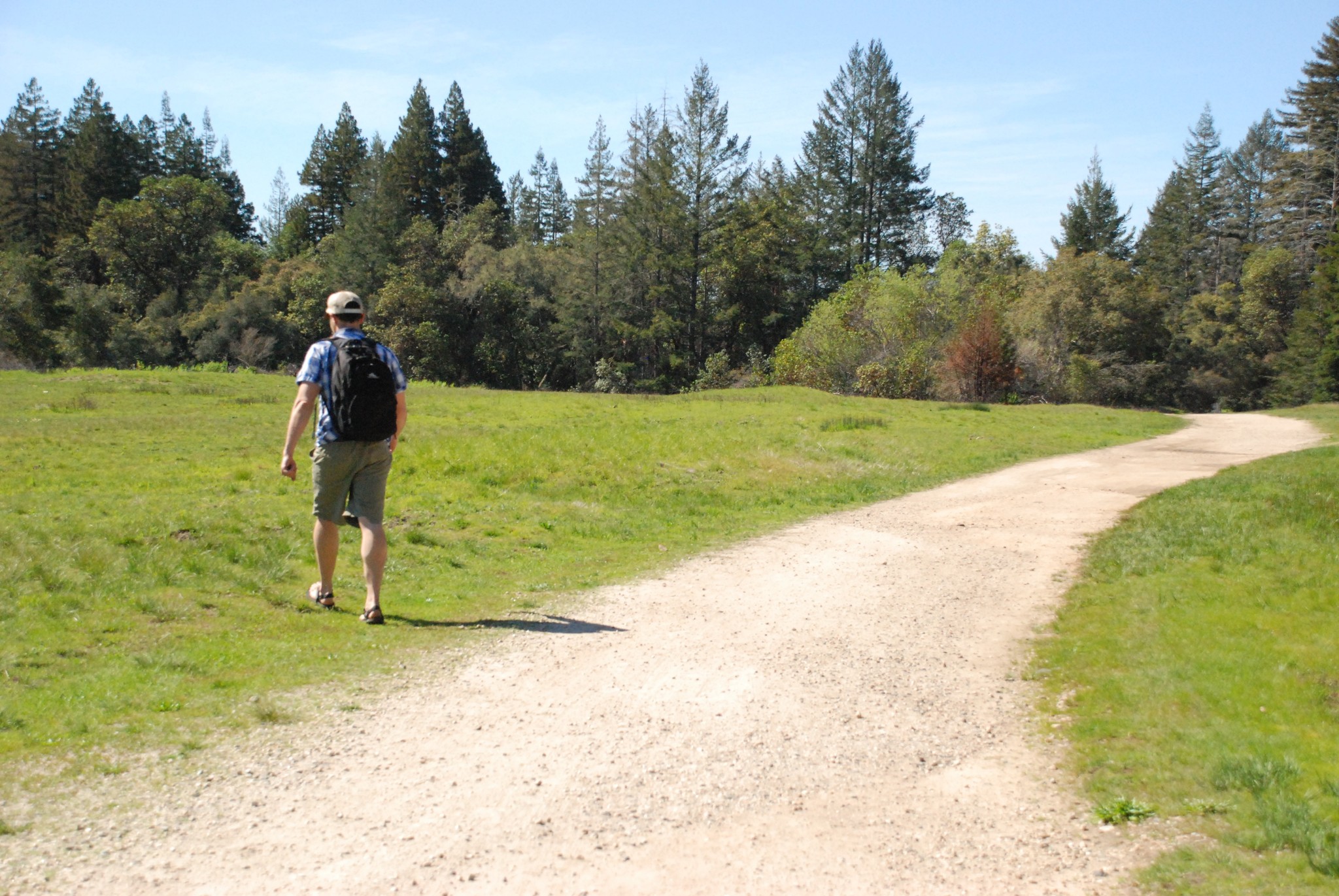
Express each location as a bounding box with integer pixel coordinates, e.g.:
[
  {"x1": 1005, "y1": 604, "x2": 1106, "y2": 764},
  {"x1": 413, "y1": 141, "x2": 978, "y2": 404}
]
[{"x1": 320, "y1": 335, "x2": 398, "y2": 441}]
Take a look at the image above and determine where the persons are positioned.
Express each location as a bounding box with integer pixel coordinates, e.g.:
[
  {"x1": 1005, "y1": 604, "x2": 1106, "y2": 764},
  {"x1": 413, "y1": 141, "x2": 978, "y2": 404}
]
[{"x1": 280, "y1": 291, "x2": 409, "y2": 623}]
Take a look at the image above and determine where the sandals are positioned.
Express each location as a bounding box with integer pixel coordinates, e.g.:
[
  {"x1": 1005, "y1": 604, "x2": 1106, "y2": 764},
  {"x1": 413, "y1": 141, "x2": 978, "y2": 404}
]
[
  {"x1": 361, "y1": 606, "x2": 384, "y2": 623},
  {"x1": 306, "y1": 587, "x2": 335, "y2": 608}
]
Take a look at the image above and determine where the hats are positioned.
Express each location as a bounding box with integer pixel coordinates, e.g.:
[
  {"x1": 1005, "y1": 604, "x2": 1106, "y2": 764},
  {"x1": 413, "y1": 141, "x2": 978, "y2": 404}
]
[{"x1": 325, "y1": 291, "x2": 363, "y2": 314}]
